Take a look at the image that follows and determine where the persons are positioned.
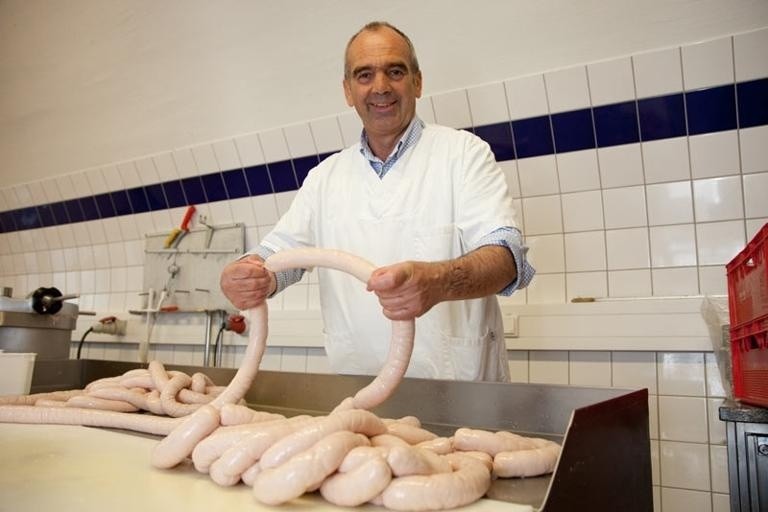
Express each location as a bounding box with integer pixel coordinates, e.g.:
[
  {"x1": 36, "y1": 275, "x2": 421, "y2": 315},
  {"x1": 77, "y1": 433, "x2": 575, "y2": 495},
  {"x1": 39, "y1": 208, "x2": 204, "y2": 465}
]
[{"x1": 220, "y1": 21, "x2": 537, "y2": 383}]
